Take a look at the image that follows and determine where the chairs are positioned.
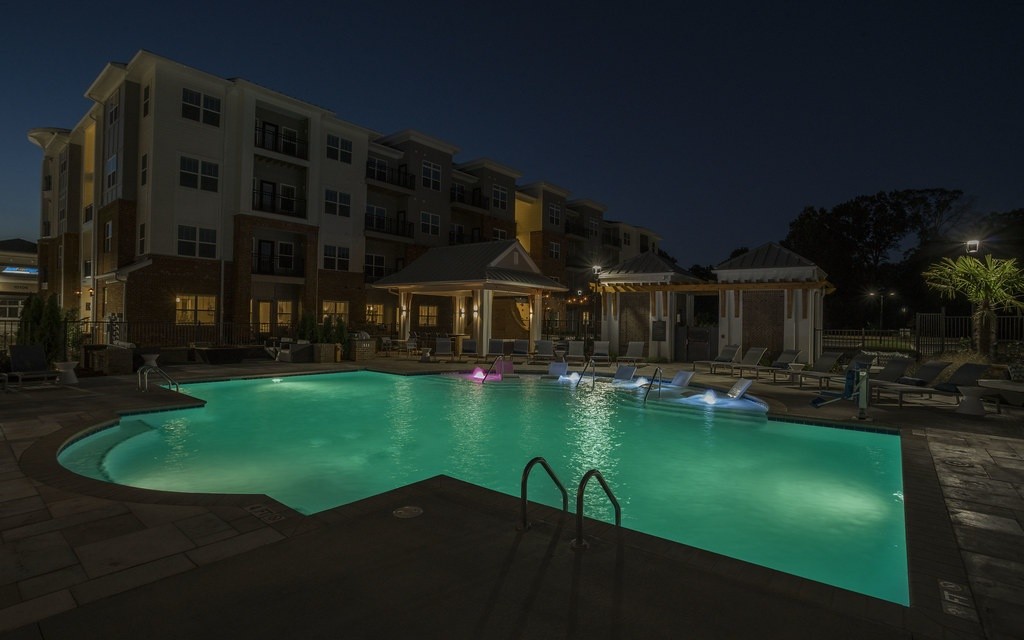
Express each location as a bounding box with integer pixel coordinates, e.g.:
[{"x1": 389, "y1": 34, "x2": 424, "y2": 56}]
[
  {"x1": 534, "y1": 340, "x2": 554, "y2": 363},
  {"x1": 589, "y1": 341, "x2": 610, "y2": 366},
  {"x1": 510, "y1": 340, "x2": 529, "y2": 365},
  {"x1": 870, "y1": 359, "x2": 992, "y2": 407},
  {"x1": 694, "y1": 344, "x2": 843, "y2": 389},
  {"x1": 486, "y1": 338, "x2": 504, "y2": 363},
  {"x1": 460, "y1": 339, "x2": 479, "y2": 363},
  {"x1": 617, "y1": 342, "x2": 645, "y2": 368},
  {"x1": 382, "y1": 337, "x2": 418, "y2": 358},
  {"x1": 435, "y1": 338, "x2": 454, "y2": 360},
  {"x1": 567, "y1": 341, "x2": 586, "y2": 365}
]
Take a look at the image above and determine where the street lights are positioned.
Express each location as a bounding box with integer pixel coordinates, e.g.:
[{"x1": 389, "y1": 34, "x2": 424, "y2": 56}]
[
  {"x1": 576, "y1": 289, "x2": 582, "y2": 340},
  {"x1": 592, "y1": 266, "x2": 602, "y2": 341},
  {"x1": 870, "y1": 292, "x2": 895, "y2": 344}
]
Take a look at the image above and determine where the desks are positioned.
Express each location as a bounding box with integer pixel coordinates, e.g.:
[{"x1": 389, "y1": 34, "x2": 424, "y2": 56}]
[
  {"x1": 142, "y1": 355, "x2": 160, "y2": 372},
  {"x1": 53, "y1": 362, "x2": 80, "y2": 384},
  {"x1": 421, "y1": 348, "x2": 432, "y2": 357},
  {"x1": 392, "y1": 340, "x2": 404, "y2": 355},
  {"x1": 789, "y1": 363, "x2": 805, "y2": 381},
  {"x1": 957, "y1": 386, "x2": 987, "y2": 415}
]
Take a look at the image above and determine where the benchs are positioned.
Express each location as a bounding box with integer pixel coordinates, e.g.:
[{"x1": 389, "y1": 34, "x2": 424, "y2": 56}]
[{"x1": 0, "y1": 344, "x2": 56, "y2": 392}]
